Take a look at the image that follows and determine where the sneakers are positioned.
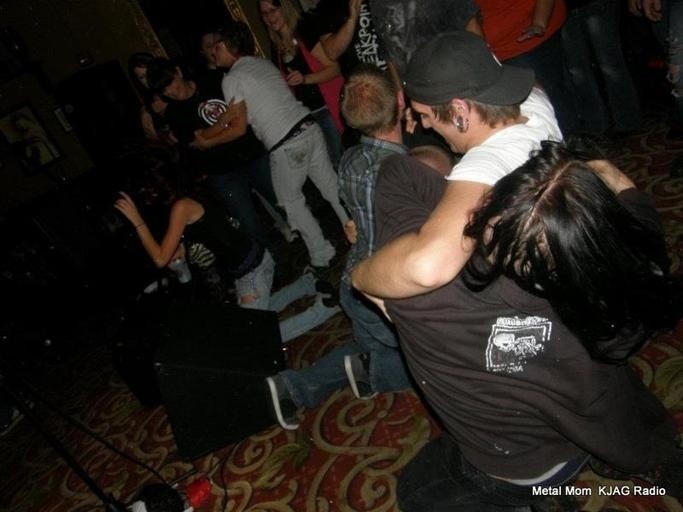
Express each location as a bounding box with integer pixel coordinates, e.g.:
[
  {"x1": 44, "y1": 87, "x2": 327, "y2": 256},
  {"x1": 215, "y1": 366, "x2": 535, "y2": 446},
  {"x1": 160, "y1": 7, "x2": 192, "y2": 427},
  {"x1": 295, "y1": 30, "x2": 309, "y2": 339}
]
[
  {"x1": 588, "y1": 445, "x2": 683, "y2": 500},
  {"x1": 266, "y1": 374, "x2": 301, "y2": 430},
  {"x1": 344, "y1": 352, "x2": 380, "y2": 401},
  {"x1": 303, "y1": 246, "x2": 343, "y2": 310},
  {"x1": 529, "y1": 493, "x2": 578, "y2": 510}
]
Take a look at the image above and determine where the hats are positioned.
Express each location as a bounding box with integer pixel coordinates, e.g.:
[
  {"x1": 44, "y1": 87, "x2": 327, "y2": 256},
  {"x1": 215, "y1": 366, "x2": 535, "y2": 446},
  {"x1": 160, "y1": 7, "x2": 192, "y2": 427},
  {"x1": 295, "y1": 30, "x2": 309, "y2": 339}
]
[{"x1": 402, "y1": 29, "x2": 534, "y2": 105}]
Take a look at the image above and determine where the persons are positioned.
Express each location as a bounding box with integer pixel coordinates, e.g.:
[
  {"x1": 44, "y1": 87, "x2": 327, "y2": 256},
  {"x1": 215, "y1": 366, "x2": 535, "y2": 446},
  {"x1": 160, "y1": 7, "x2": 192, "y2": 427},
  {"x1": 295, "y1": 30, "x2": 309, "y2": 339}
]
[{"x1": 1, "y1": 1, "x2": 683, "y2": 512}]
